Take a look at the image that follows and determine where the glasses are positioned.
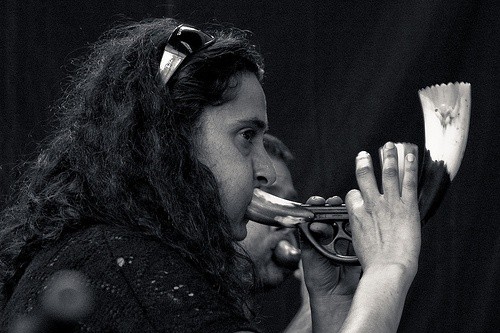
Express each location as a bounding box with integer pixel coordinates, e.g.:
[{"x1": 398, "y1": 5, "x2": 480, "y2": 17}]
[{"x1": 158, "y1": 24, "x2": 215, "y2": 86}]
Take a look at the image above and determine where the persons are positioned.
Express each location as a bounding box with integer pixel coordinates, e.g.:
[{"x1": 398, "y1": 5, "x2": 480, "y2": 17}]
[
  {"x1": 2, "y1": 16, "x2": 423, "y2": 332},
  {"x1": 228, "y1": 131, "x2": 313, "y2": 333}
]
